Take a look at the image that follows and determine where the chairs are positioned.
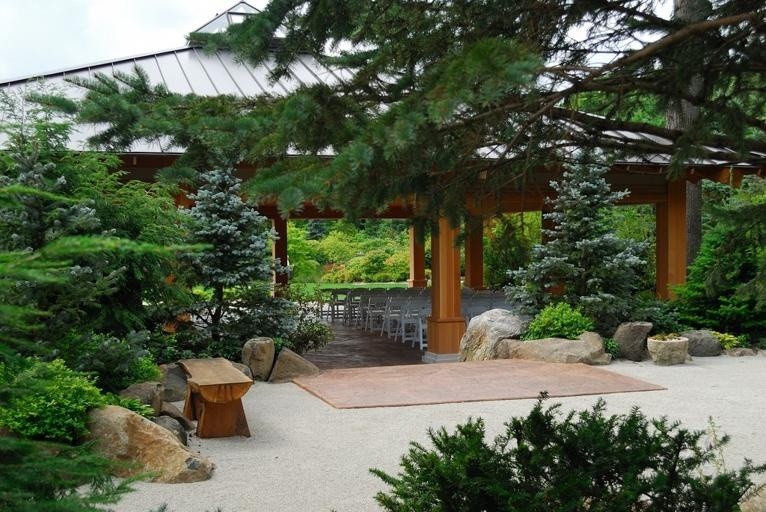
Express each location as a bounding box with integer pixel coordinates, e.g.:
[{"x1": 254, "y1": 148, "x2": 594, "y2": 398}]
[{"x1": 315, "y1": 285, "x2": 521, "y2": 352}]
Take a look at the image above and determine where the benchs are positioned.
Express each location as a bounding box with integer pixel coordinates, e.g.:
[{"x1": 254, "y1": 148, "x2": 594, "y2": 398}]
[{"x1": 175, "y1": 357, "x2": 256, "y2": 439}]
